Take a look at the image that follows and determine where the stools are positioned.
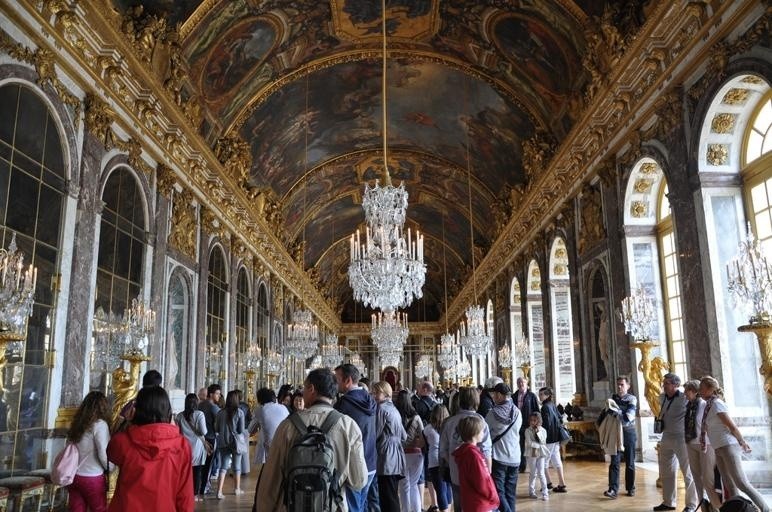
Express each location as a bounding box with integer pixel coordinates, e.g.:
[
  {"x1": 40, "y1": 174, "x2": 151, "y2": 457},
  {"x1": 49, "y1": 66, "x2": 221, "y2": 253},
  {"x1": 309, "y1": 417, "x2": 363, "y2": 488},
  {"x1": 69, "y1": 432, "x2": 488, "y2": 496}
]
[
  {"x1": 1, "y1": 473, "x2": 47, "y2": 512},
  {"x1": 25, "y1": 469, "x2": 72, "y2": 512},
  {"x1": 1, "y1": 483, "x2": 11, "y2": 512}
]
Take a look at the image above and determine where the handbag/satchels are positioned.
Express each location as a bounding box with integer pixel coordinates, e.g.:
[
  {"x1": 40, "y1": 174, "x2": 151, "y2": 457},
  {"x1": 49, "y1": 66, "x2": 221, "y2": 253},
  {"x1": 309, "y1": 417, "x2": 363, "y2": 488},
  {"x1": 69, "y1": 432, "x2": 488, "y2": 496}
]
[
  {"x1": 558, "y1": 425, "x2": 572, "y2": 446},
  {"x1": 229, "y1": 433, "x2": 247, "y2": 452},
  {"x1": 376, "y1": 431, "x2": 390, "y2": 455},
  {"x1": 441, "y1": 459, "x2": 450, "y2": 482},
  {"x1": 700, "y1": 498, "x2": 717, "y2": 512},
  {"x1": 51, "y1": 441, "x2": 80, "y2": 487},
  {"x1": 205, "y1": 440, "x2": 216, "y2": 456},
  {"x1": 421, "y1": 397, "x2": 438, "y2": 419}
]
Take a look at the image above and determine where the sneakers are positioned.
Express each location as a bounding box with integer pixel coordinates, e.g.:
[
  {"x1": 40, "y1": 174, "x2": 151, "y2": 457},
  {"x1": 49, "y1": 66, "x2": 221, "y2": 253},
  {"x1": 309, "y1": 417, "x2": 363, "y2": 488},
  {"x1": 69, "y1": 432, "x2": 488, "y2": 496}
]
[
  {"x1": 543, "y1": 494, "x2": 549, "y2": 501},
  {"x1": 427, "y1": 505, "x2": 438, "y2": 511},
  {"x1": 194, "y1": 486, "x2": 245, "y2": 503},
  {"x1": 531, "y1": 493, "x2": 538, "y2": 499}
]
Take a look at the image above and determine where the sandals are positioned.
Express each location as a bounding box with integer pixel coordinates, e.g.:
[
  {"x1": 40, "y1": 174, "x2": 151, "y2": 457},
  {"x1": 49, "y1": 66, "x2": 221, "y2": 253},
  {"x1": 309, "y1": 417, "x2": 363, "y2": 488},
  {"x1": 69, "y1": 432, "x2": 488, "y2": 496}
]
[
  {"x1": 539, "y1": 482, "x2": 552, "y2": 491},
  {"x1": 552, "y1": 485, "x2": 566, "y2": 492}
]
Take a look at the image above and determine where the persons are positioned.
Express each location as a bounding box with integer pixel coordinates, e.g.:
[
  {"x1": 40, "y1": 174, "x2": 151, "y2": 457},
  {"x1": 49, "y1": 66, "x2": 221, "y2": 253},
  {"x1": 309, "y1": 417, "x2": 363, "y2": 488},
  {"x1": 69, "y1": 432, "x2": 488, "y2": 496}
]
[
  {"x1": 682, "y1": 378, "x2": 723, "y2": 511},
  {"x1": 2, "y1": 367, "x2": 34, "y2": 452},
  {"x1": 332, "y1": 364, "x2": 570, "y2": 512},
  {"x1": 176, "y1": 369, "x2": 304, "y2": 502},
  {"x1": 604, "y1": 375, "x2": 638, "y2": 499},
  {"x1": 121, "y1": 370, "x2": 162, "y2": 420},
  {"x1": 64, "y1": 392, "x2": 114, "y2": 512},
  {"x1": 107, "y1": 384, "x2": 196, "y2": 512},
  {"x1": 698, "y1": 374, "x2": 771, "y2": 512},
  {"x1": 651, "y1": 373, "x2": 697, "y2": 511},
  {"x1": 632, "y1": 355, "x2": 668, "y2": 425},
  {"x1": 256, "y1": 367, "x2": 368, "y2": 512}
]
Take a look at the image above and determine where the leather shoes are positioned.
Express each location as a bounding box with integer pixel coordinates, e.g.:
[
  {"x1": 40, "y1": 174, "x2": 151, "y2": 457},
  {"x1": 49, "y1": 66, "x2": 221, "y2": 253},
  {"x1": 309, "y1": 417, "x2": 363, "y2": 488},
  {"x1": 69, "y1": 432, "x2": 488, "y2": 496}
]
[
  {"x1": 604, "y1": 489, "x2": 617, "y2": 500},
  {"x1": 682, "y1": 506, "x2": 698, "y2": 512},
  {"x1": 628, "y1": 487, "x2": 636, "y2": 496},
  {"x1": 653, "y1": 503, "x2": 676, "y2": 511}
]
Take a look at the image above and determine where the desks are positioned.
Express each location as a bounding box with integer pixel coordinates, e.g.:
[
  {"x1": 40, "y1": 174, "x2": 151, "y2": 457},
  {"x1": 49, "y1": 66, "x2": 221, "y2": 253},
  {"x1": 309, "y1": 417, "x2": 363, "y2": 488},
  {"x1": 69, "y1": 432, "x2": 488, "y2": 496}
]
[{"x1": 559, "y1": 419, "x2": 604, "y2": 459}]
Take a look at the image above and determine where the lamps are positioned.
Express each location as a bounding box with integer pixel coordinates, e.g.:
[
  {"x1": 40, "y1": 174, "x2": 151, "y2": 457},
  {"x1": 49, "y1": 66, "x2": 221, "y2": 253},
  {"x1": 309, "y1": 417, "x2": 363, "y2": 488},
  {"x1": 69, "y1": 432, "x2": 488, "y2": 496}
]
[
  {"x1": 414, "y1": 352, "x2": 434, "y2": 378},
  {"x1": 285, "y1": 108, "x2": 319, "y2": 352},
  {"x1": 322, "y1": 217, "x2": 342, "y2": 367},
  {"x1": 1, "y1": 228, "x2": 41, "y2": 413},
  {"x1": 436, "y1": 209, "x2": 460, "y2": 368},
  {"x1": 108, "y1": 294, "x2": 157, "y2": 435},
  {"x1": 443, "y1": 359, "x2": 471, "y2": 381},
  {"x1": 369, "y1": 312, "x2": 410, "y2": 372},
  {"x1": 243, "y1": 281, "x2": 263, "y2": 369},
  {"x1": 348, "y1": 2, "x2": 428, "y2": 310},
  {"x1": 459, "y1": 130, "x2": 492, "y2": 357},
  {"x1": 498, "y1": 312, "x2": 514, "y2": 368},
  {"x1": 723, "y1": 218, "x2": 772, "y2": 407},
  {"x1": 349, "y1": 312, "x2": 368, "y2": 378},
  {"x1": 619, "y1": 283, "x2": 672, "y2": 420},
  {"x1": 263, "y1": 307, "x2": 284, "y2": 375},
  {"x1": 514, "y1": 294, "x2": 532, "y2": 365}
]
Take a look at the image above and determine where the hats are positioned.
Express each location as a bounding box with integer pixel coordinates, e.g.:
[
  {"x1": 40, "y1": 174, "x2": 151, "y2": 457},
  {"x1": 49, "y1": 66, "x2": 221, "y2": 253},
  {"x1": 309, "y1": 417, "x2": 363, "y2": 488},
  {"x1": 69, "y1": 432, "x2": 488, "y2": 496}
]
[{"x1": 487, "y1": 384, "x2": 513, "y2": 396}]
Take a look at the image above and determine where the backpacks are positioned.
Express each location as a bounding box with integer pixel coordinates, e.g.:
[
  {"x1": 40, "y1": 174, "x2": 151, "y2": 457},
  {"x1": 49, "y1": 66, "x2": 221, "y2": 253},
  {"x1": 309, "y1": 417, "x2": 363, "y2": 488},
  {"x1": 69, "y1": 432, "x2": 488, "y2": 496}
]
[
  {"x1": 283, "y1": 409, "x2": 346, "y2": 512},
  {"x1": 720, "y1": 497, "x2": 759, "y2": 512},
  {"x1": 405, "y1": 419, "x2": 426, "y2": 448}
]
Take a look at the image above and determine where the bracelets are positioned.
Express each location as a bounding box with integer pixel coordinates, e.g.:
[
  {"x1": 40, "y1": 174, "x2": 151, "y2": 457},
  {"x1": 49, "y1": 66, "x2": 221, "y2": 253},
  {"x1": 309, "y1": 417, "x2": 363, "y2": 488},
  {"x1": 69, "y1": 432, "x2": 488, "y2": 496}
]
[{"x1": 738, "y1": 439, "x2": 745, "y2": 445}]
